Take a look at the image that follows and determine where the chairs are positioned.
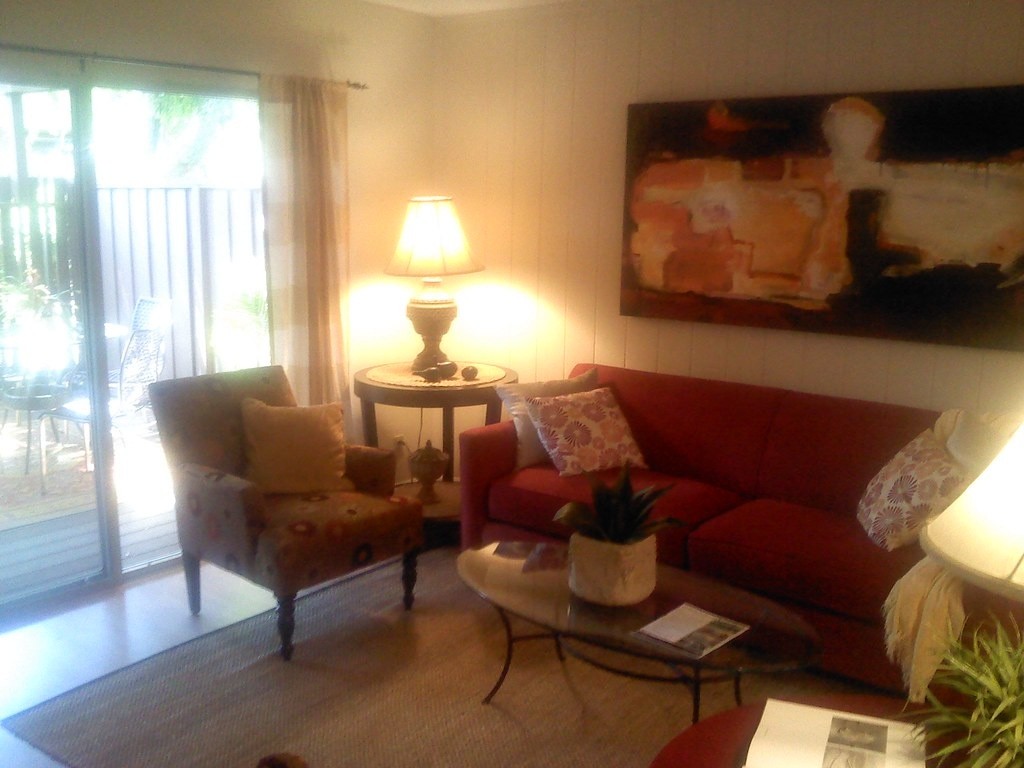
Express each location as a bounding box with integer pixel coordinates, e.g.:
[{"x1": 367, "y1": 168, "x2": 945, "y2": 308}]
[
  {"x1": 106, "y1": 298, "x2": 175, "y2": 422},
  {"x1": 32, "y1": 327, "x2": 164, "y2": 497}
]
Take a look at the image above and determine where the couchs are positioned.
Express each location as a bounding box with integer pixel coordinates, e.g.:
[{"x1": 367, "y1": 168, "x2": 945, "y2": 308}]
[
  {"x1": 142, "y1": 363, "x2": 420, "y2": 666},
  {"x1": 457, "y1": 360, "x2": 1024, "y2": 711}
]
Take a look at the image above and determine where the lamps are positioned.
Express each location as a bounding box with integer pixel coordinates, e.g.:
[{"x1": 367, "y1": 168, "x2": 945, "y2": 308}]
[
  {"x1": 913, "y1": 417, "x2": 1024, "y2": 627},
  {"x1": 380, "y1": 189, "x2": 489, "y2": 383}
]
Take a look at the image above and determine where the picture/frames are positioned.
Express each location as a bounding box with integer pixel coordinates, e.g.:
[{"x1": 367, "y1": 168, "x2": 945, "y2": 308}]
[{"x1": 616, "y1": 85, "x2": 1024, "y2": 356}]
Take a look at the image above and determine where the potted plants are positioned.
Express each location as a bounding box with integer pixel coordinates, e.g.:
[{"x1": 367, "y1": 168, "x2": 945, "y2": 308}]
[{"x1": 550, "y1": 460, "x2": 679, "y2": 611}]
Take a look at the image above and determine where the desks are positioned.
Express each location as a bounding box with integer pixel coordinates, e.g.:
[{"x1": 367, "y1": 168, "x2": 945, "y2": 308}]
[
  {"x1": 104, "y1": 320, "x2": 131, "y2": 371},
  {"x1": 0, "y1": 384, "x2": 81, "y2": 476}
]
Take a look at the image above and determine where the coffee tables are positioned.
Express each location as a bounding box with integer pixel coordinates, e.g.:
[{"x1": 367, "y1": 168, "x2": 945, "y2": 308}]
[{"x1": 453, "y1": 540, "x2": 830, "y2": 725}]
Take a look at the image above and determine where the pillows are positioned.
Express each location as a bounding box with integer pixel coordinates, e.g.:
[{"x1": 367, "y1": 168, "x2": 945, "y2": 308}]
[
  {"x1": 853, "y1": 424, "x2": 983, "y2": 558},
  {"x1": 522, "y1": 386, "x2": 646, "y2": 485},
  {"x1": 233, "y1": 395, "x2": 358, "y2": 498},
  {"x1": 490, "y1": 367, "x2": 601, "y2": 472}
]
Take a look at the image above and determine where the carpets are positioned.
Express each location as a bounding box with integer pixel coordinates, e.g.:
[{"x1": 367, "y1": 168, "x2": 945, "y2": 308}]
[{"x1": 0, "y1": 545, "x2": 1024, "y2": 768}]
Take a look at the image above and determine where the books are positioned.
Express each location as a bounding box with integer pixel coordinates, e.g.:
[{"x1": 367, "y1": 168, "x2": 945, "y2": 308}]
[
  {"x1": 740, "y1": 695, "x2": 927, "y2": 768},
  {"x1": 637, "y1": 601, "x2": 750, "y2": 656}
]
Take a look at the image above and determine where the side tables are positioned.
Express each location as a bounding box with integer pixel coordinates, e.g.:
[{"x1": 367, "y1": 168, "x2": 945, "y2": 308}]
[
  {"x1": 644, "y1": 688, "x2": 970, "y2": 768},
  {"x1": 350, "y1": 360, "x2": 521, "y2": 551}
]
